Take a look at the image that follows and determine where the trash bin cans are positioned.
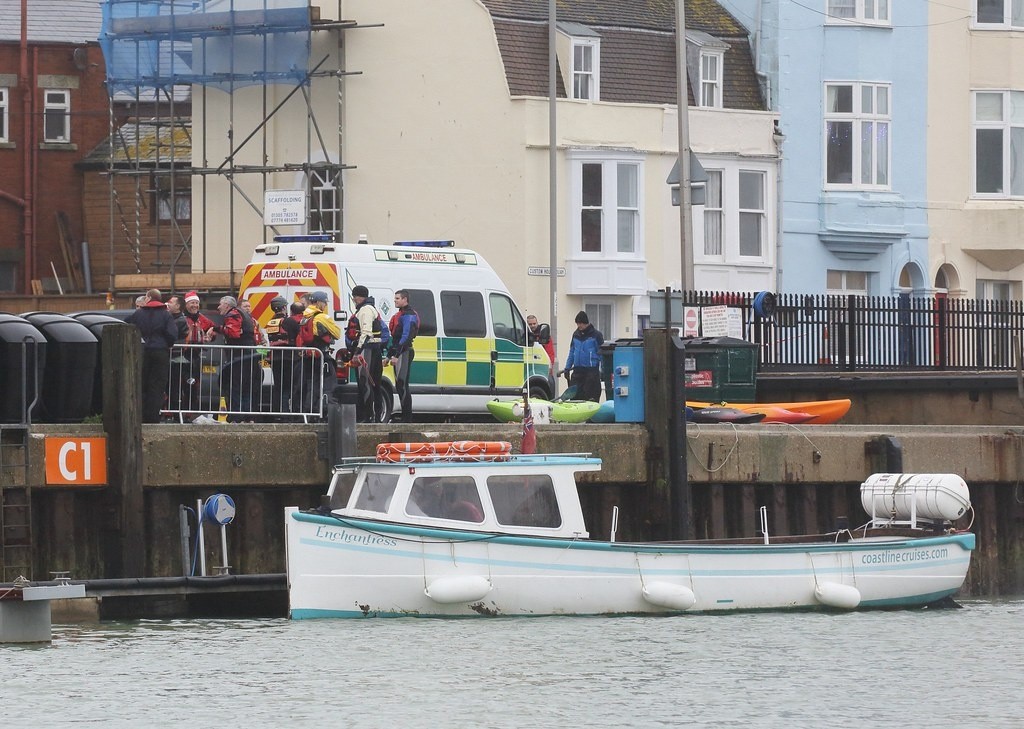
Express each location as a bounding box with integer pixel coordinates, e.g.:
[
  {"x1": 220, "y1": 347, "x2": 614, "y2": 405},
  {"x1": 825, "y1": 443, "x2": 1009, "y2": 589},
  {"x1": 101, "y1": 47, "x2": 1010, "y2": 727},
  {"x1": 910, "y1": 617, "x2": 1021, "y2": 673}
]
[
  {"x1": 601, "y1": 338, "x2": 645, "y2": 423},
  {"x1": 681, "y1": 335, "x2": 757, "y2": 404}
]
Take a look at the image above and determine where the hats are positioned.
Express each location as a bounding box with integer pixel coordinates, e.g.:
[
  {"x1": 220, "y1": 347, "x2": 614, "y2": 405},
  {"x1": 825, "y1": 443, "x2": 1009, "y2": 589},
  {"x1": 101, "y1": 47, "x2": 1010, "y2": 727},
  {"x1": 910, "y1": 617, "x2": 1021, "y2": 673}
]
[
  {"x1": 310, "y1": 291, "x2": 330, "y2": 303},
  {"x1": 184, "y1": 292, "x2": 200, "y2": 303},
  {"x1": 575, "y1": 311, "x2": 589, "y2": 324},
  {"x1": 271, "y1": 297, "x2": 288, "y2": 311},
  {"x1": 352, "y1": 286, "x2": 368, "y2": 297}
]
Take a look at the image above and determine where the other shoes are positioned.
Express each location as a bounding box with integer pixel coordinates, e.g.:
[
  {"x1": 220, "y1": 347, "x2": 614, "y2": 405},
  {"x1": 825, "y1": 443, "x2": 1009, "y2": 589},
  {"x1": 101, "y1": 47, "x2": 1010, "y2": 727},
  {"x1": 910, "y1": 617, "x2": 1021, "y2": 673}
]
[{"x1": 359, "y1": 418, "x2": 373, "y2": 423}]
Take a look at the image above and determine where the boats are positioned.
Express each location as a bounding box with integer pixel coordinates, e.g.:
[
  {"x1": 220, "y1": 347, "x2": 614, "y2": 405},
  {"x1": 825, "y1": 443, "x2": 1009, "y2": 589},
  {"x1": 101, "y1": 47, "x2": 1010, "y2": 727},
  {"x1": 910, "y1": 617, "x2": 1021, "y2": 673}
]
[
  {"x1": 586, "y1": 400, "x2": 614, "y2": 423},
  {"x1": 284, "y1": 453, "x2": 977, "y2": 622},
  {"x1": 686, "y1": 406, "x2": 820, "y2": 424},
  {"x1": 686, "y1": 399, "x2": 852, "y2": 424},
  {"x1": 486, "y1": 396, "x2": 603, "y2": 423}
]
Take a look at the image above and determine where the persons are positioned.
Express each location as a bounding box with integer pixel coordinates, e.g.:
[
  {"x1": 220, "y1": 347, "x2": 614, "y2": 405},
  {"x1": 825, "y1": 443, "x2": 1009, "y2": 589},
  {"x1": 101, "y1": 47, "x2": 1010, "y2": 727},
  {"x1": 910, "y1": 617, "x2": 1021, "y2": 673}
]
[
  {"x1": 124, "y1": 289, "x2": 217, "y2": 423},
  {"x1": 206, "y1": 296, "x2": 270, "y2": 423},
  {"x1": 564, "y1": 311, "x2": 604, "y2": 401},
  {"x1": 527, "y1": 315, "x2": 555, "y2": 365},
  {"x1": 264, "y1": 291, "x2": 341, "y2": 421},
  {"x1": 345, "y1": 285, "x2": 390, "y2": 423},
  {"x1": 382, "y1": 291, "x2": 420, "y2": 423}
]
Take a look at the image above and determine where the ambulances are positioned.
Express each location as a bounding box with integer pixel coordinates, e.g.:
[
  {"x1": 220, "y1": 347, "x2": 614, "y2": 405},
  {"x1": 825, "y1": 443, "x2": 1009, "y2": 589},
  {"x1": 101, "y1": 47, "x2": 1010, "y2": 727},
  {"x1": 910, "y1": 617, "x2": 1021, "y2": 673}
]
[{"x1": 235, "y1": 231, "x2": 554, "y2": 423}]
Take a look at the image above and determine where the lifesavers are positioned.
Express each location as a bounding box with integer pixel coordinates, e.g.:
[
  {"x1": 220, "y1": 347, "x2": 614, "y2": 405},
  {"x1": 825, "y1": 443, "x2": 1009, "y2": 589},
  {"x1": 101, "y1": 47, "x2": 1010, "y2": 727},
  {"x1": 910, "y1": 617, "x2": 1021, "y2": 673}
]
[
  {"x1": 376, "y1": 441, "x2": 513, "y2": 452},
  {"x1": 375, "y1": 453, "x2": 513, "y2": 462}
]
